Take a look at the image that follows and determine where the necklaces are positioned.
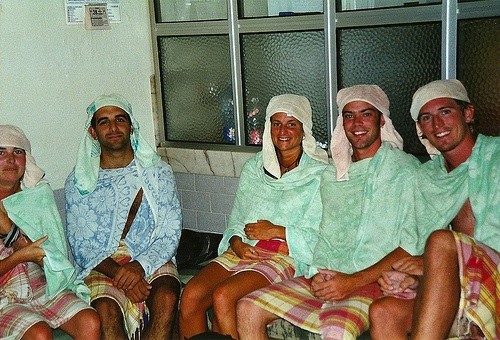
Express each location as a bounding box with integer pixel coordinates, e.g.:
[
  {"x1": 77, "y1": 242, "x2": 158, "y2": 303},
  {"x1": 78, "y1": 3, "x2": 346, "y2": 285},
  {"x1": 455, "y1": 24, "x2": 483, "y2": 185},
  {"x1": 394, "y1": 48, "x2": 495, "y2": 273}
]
[{"x1": 276, "y1": 156, "x2": 297, "y2": 174}]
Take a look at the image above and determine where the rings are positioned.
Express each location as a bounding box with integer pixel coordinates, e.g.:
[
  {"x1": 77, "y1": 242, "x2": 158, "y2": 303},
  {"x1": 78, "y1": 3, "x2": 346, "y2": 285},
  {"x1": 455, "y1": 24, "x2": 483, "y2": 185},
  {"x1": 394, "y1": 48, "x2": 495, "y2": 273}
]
[
  {"x1": 249, "y1": 234, "x2": 251, "y2": 238},
  {"x1": 248, "y1": 227, "x2": 250, "y2": 231},
  {"x1": 252, "y1": 250, "x2": 255, "y2": 254}
]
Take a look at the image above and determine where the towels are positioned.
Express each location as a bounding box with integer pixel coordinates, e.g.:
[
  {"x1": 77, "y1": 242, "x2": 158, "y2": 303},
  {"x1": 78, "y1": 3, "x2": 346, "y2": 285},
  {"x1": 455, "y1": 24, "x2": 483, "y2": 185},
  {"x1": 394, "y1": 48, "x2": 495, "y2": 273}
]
[{"x1": 0, "y1": 77, "x2": 500, "y2": 340}]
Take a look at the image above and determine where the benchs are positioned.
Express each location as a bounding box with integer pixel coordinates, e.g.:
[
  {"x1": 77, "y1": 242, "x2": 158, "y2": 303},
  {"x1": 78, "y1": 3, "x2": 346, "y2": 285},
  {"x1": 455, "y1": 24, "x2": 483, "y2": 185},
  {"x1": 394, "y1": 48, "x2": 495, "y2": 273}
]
[{"x1": 52, "y1": 265, "x2": 374, "y2": 340}]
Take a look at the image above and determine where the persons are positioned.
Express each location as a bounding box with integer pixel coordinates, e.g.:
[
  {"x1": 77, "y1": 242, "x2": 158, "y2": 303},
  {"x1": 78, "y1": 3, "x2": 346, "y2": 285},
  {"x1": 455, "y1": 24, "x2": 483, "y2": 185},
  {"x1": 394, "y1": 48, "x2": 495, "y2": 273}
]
[
  {"x1": 179, "y1": 94, "x2": 322, "y2": 340},
  {"x1": 236, "y1": 84, "x2": 422, "y2": 340},
  {"x1": 369, "y1": 80, "x2": 500, "y2": 340},
  {"x1": 65, "y1": 94, "x2": 182, "y2": 340},
  {"x1": 0, "y1": 125, "x2": 101, "y2": 340}
]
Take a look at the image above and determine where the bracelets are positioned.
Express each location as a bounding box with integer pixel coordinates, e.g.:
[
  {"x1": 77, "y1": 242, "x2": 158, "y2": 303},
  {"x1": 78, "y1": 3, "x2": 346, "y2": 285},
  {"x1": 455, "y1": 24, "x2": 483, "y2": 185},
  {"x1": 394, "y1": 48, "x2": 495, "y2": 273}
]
[{"x1": 3, "y1": 222, "x2": 28, "y2": 248}]
